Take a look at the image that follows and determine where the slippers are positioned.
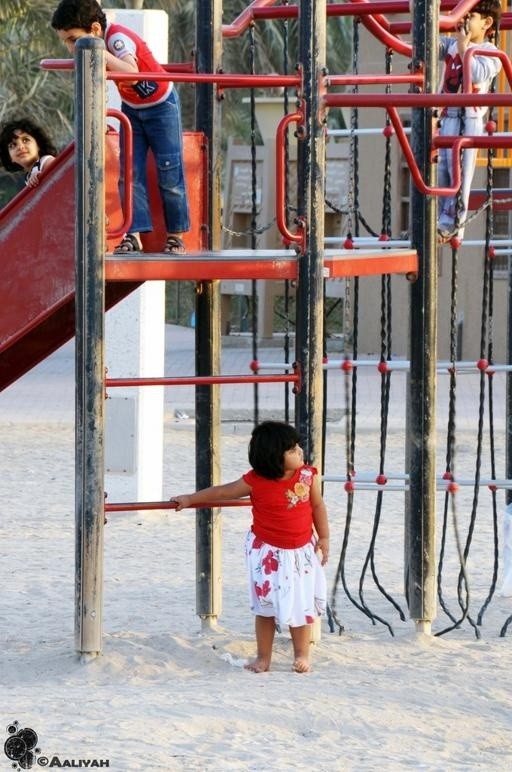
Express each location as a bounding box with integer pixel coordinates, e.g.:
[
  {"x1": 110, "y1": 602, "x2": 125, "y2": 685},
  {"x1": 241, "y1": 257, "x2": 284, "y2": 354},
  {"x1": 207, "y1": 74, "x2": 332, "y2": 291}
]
[
  {"x1": 113, "y1": 232, "x2": 144, "y2": 254},
  {"x1": 165, "y1": 235, "x2": 186, "y2": 255}
]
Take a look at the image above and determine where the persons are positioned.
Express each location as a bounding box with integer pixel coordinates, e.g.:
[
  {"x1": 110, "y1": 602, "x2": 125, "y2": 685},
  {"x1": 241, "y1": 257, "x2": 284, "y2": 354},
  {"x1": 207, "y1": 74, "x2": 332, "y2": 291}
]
[
  {"x1": 239, "y1": 295, "x2": 249, "y2": 332},
  {"x1": 51, "y1": 0, "x2": 191, "y2": 256},
  {"x1": 170, "y1": 419, "x2": 330, "y2": 673},
  {"x1": 397, "y1": 0, "x2": 503, "y2": 245},
  {"x1": 0, "y1": 116, "x2": 58, "y2": 188}
]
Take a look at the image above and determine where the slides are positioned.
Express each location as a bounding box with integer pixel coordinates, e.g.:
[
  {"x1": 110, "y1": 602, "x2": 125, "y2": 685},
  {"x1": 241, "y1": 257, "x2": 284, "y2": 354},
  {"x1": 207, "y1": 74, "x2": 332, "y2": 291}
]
[{"x1": 0, "y1": 124, "x2": 208, "y2": 392}]
